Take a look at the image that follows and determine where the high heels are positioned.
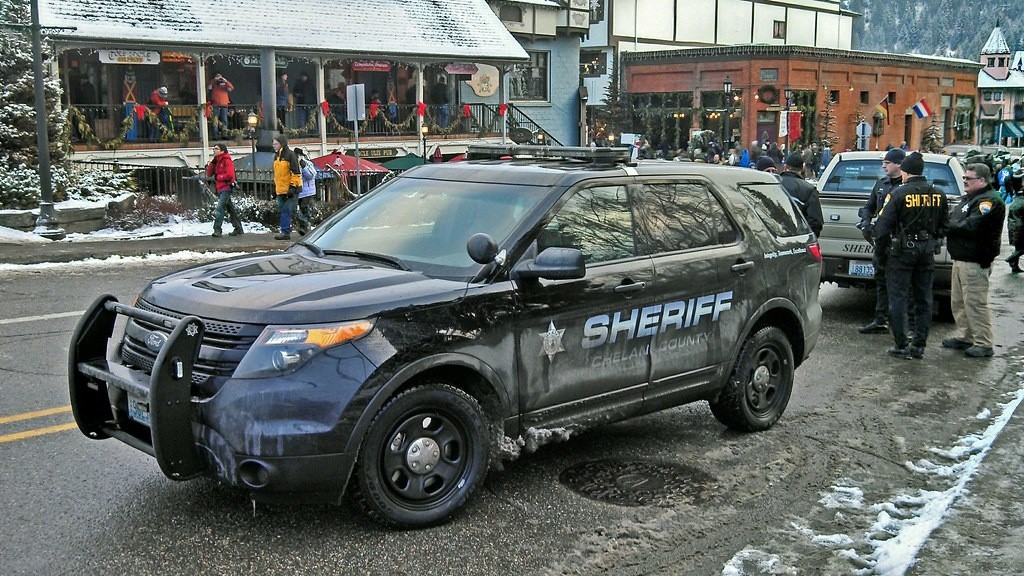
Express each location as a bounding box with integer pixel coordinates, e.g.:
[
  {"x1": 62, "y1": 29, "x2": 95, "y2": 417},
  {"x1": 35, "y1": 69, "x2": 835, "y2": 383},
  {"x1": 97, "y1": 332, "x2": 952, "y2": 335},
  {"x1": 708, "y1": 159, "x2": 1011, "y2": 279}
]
[{"x1": 1004, "y1": 252, "x2": 1024, "y2": 273}]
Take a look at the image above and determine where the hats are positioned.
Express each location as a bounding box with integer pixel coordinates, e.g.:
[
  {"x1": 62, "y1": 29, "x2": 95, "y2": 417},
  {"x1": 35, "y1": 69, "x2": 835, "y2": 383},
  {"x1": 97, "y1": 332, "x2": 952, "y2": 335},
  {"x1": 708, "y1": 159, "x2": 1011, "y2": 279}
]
[
  {"x1": 900, "y1": 151, "x2": 924, "y2": 176},
  {"x1": 884, "y1": 148, "x2": 905, "y2": 164},
  {"x1": 159, "y1": 86, "x2": 168, "y2": 97},
  {"x1": 755, "y1": 155, "x2": 776, "y2": 170},
  {"x1": 272, "y1": 134, "x2": 287, "y2": 148},
  {"x1": 787, "y1": 152, "x2": 804, "y2": 168}
]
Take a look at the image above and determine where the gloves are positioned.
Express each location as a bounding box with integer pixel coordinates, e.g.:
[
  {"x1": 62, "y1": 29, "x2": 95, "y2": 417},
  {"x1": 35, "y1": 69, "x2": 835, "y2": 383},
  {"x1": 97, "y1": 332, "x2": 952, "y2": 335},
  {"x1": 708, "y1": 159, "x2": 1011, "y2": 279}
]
[{"x1": 300, "y1": 159, "x2": 306, "y2": 168}]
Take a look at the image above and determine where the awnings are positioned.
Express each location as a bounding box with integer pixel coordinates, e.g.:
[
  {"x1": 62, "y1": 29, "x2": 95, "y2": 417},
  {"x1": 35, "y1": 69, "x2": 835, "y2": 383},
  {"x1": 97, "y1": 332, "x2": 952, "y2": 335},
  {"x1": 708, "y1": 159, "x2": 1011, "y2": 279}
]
[
  {"x1": 1001, "y1": 121, "x2": 1024, "y2": 139},
  {"x1": 1014, "y1": 121, "x2": 1024, "y2": 135}
]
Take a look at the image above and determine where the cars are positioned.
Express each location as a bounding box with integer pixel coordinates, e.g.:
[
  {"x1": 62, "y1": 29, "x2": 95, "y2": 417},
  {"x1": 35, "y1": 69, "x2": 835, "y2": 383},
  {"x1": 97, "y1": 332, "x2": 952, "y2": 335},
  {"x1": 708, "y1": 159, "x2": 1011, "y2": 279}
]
[{"x1": 939, "y1": 145, "x2": 1024, "y2": 175}]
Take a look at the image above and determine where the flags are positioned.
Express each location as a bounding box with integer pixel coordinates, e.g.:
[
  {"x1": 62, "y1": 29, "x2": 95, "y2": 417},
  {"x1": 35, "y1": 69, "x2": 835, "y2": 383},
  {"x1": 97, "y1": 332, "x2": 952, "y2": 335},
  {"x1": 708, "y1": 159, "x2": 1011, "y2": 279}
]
[
  {"x1": 912, "y1": 98, "x2": 932, "y2": 120},
  {"x1": 874, "y1": 97, "x2": 890, "y2": 126}
]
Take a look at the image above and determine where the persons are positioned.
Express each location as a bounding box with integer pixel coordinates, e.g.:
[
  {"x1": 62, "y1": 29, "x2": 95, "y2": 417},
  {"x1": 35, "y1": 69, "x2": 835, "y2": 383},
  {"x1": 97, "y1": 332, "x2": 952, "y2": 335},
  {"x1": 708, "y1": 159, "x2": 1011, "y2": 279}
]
[
  {"x1": 271, "y1": 133, "x2": 312, "y2": 241},
  {"x1": 206, "y1": 143, "x2": 245, "y2": 237},
  {"x1": 293, "y1": 147, "x2": 317, "y2": 237},
  {"x1": 58, "y1": 64, "x2": 454, "y2": 144},
  {"x1": 777, "y1": 153, "x2": 825, "y2": 239},
  {"x1": 855, "y1": 147, "x2": 915, "y2": 342},
  {"x1": 585, "y1": 127, "x2": 836, "y2": 187},
  {"x1": 945, "y1": 162, "x2": 1006, "y2": 357},
  {"x1": 844, "y1": 141, "x2": 1024, "y2": 273},
  {"x1": 868, "y1": 151, "x2": 949, "y2": 361}
]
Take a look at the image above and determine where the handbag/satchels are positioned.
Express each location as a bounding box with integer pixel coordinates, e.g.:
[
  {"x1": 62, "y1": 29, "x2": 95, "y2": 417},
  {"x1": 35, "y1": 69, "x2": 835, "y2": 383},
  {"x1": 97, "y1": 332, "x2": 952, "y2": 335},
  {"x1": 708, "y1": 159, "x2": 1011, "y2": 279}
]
[{"x1": 209, "y1": 174, "x2": 219, "y2": 197}]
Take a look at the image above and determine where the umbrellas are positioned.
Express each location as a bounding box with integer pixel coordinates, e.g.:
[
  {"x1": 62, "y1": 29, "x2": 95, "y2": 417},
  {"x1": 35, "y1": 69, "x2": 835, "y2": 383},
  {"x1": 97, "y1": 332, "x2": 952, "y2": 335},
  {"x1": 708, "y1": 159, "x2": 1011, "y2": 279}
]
[
  {"x1": 378, "y1": 152, "x2": 434, "y2": 174},
  {"x1": 447, "y1": 153, "x2": 514, "y2": 162},
  {"x1": 307, "y1": 148, "x2": 391, "y2": 177}
]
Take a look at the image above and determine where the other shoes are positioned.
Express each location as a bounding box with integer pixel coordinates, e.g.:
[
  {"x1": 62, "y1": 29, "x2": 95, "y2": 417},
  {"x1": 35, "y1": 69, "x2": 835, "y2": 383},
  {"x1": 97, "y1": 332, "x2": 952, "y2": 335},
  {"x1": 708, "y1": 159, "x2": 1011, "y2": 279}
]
[
  {"x1": 941, "y1": 337, "x2": 972, "y2": 349},
  {"x1": 889, "y1": 343, "x2": 925, "y2": 359},
  {"x1": 905, "y1": 330, "x2": 914, "y2": 342},
  {"x1": 965, "y1": 346, "x2": 994, "y2": 357},
  {"x1": 212, "y1": 232, "x2": 223, "y2": 237},
  {"x1": 275, "y1": 234, "x2": 291, "y2": 240},
  {"x1": 229, "y1": 229, "x2": 241, "y2": 236},
  {"x1": 858, "y1": 321, "x2": 890, "y2": 334}
]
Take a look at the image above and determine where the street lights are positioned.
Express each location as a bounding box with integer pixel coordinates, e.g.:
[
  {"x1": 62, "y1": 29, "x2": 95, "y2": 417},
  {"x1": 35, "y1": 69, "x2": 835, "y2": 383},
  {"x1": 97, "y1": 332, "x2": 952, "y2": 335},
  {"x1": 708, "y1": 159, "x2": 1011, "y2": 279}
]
[
  {"x1": 782, "y1": 86, "x2": 794, "y2": 164},
  {"x1": 722, "y1": 74, "x2": 733, "y2": 160}
]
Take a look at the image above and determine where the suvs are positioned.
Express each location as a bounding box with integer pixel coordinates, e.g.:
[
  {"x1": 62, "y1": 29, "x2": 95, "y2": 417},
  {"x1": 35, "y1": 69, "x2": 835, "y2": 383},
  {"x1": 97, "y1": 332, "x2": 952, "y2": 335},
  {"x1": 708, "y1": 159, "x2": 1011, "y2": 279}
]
[
  {"x1": 66, "y1": 138, "x2": 827, "y2": 533},
  {"x1": 814, "y1": 150, "x2": 970, "y2": 324}
]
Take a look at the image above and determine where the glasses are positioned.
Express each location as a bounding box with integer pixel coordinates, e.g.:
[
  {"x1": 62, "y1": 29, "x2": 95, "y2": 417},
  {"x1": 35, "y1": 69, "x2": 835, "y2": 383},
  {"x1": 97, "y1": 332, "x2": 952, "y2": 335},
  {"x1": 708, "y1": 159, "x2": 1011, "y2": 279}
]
[
  {"x1": 767, "y1": 170, "x2": 776, "y2": 173},
  {"x1": 882, "y1": 161, "x2": 891, "y2": 165},
  {"x1": 962, "y1": 175, "x2": 981, "y2": 180}
]
